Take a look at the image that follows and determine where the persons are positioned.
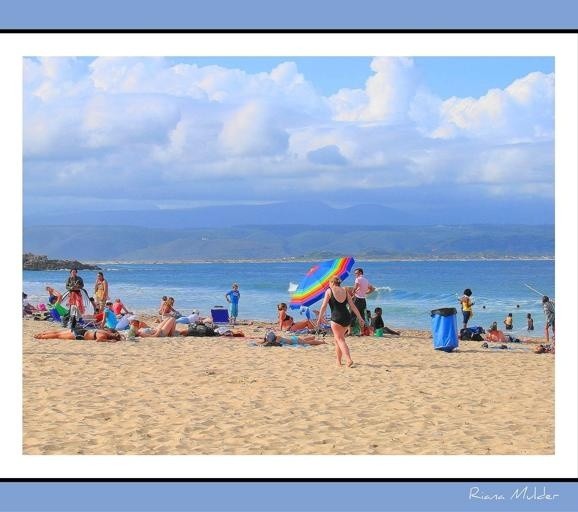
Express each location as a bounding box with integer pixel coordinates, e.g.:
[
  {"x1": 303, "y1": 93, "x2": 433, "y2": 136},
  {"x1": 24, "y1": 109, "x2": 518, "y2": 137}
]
[
  {"x1": 276, "y1": 268, "x2": 400, "y2": 336},
  {"x1": 225, "y1": 283, "x2": 240, "y2": 324},
  {"x1": 22, "y1": 267, "x2": 212, "y2": 342},
  {"x1": 316, "y1": 278, "x2": 365, "y2": 367},
  {"x1": 459, "y1": 288, "x2": 555, "y2": 343}
]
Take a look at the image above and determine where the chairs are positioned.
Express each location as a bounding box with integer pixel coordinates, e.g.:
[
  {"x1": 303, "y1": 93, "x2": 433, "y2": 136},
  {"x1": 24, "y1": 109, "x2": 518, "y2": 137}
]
[{"x1": 211, "y1": 305, "x2": 234, "y2": 330}]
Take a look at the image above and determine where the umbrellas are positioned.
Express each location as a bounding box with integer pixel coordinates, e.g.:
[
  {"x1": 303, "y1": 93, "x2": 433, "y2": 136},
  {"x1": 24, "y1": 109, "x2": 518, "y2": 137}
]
[{"x1": 288, "y1": 255, "x2": 355, "y2": 310}]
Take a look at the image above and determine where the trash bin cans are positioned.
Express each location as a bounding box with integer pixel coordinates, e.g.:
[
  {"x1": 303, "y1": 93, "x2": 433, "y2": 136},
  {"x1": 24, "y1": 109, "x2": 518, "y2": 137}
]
[{"x1": 431, "y1": 308, "x2": 458, "y2": 353}]
